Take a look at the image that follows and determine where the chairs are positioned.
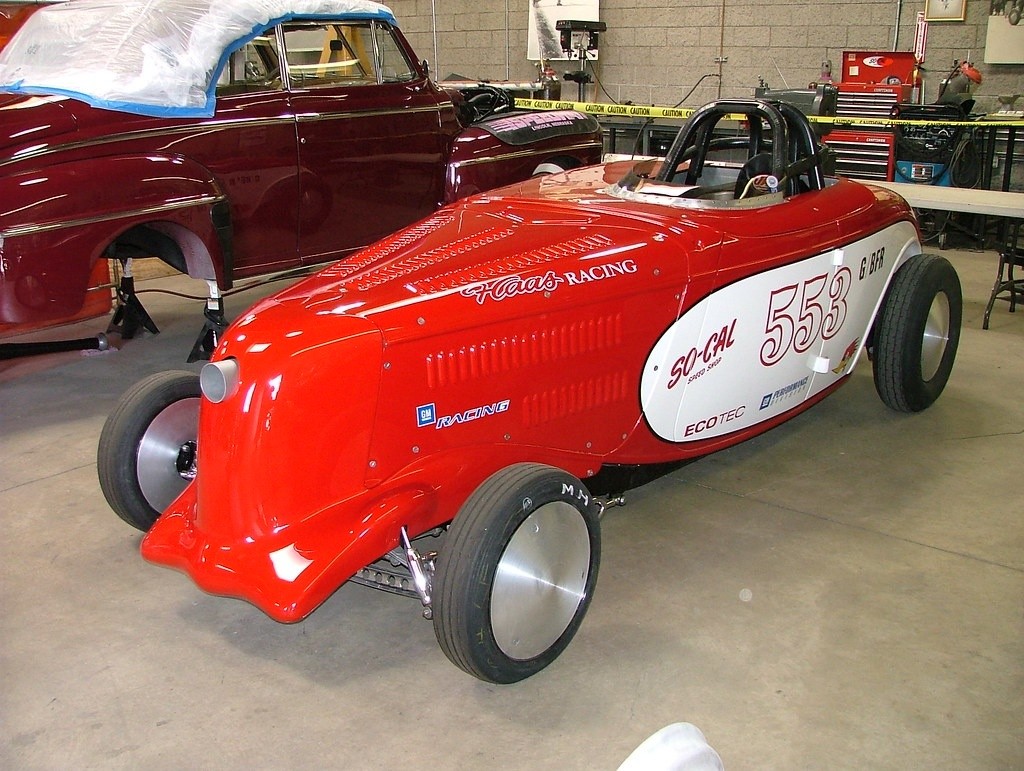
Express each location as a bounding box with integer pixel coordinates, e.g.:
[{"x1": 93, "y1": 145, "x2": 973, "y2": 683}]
[
  {"x1": 983, "y1": 219, "x2": 1024, "y2": 331},
  {"x1": 733, "y1": 153, "x2": 800, "y2": 200}
]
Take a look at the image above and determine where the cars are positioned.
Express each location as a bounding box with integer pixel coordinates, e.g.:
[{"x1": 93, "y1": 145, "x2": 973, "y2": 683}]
[
  {"x1": 96, "y1": 96, "x2": 964, "y2": 684},
  {"x1": 0, "y1": 0, "x2": 604, "y2": 353}
]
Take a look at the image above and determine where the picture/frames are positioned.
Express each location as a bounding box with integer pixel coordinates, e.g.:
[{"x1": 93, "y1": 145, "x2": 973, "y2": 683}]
[{"x1": 924, "y1": 0, "x2": 967, "y2": 22}]
